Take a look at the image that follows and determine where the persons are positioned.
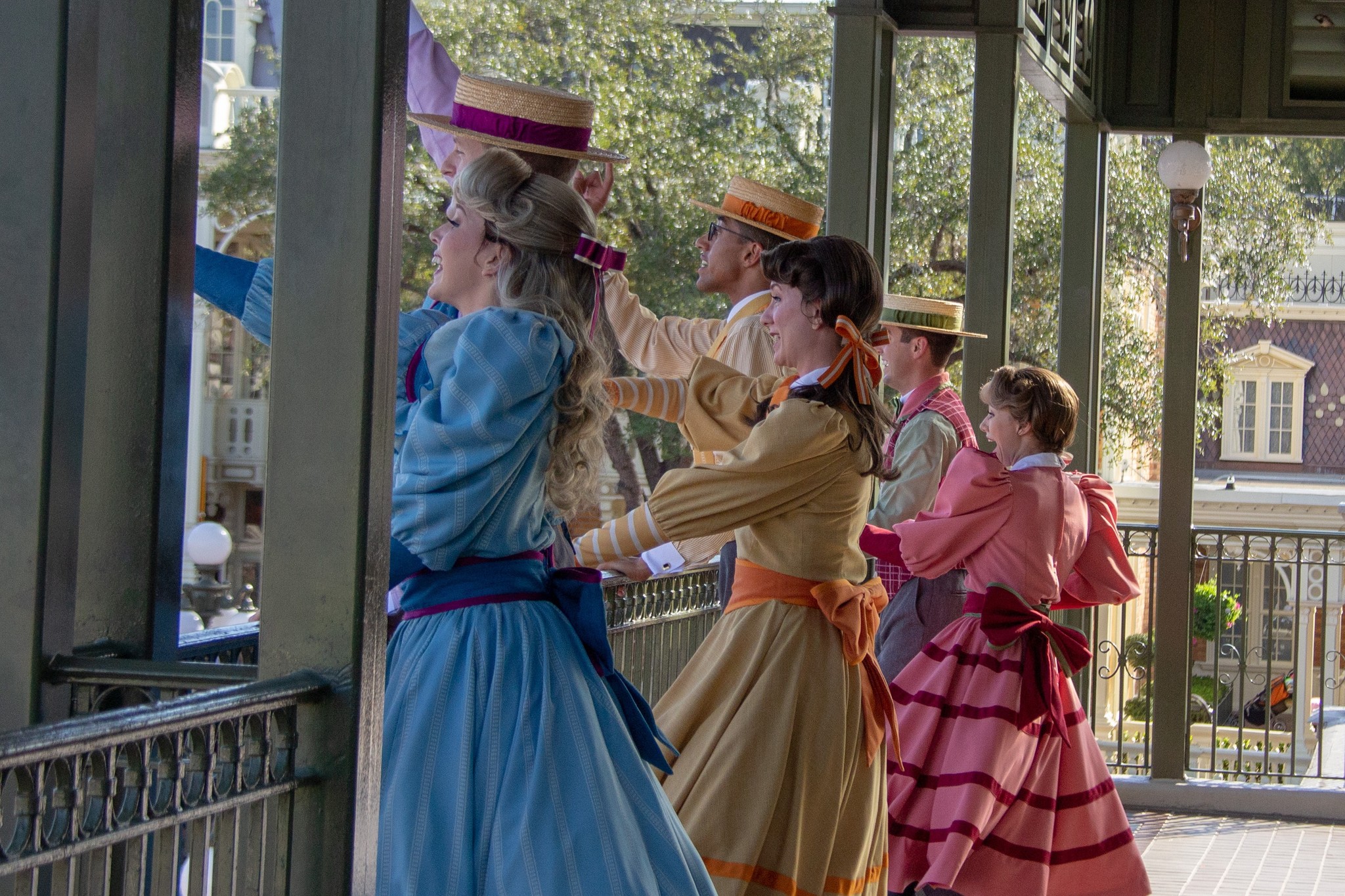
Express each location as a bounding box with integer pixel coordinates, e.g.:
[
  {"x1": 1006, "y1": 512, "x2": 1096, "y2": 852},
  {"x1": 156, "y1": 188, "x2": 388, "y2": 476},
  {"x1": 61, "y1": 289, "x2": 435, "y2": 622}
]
[
  {"x1": 570, "y1": 162, "x2": 827, "y2": 617},
  {"x1": 862, "y1": 293, "x2": 988, "y2": 686},
  {"x1": 406, "y1": 0, "x2": 631, "y2": 192},
  {"x1": 856, "y1": 360, "x2": 1153, "y2": 896},
  {"x1": 193, "y1": 145, "x2": 721, "y2": 896},
  {"x1": 568, "y1": 234, "x2": 907, "y2": 895}
]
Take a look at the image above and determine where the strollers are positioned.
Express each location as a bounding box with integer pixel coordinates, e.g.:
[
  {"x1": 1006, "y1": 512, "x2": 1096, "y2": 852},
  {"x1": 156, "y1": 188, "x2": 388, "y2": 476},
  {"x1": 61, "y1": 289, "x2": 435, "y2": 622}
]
[{"x1": 1229, "y1": 669, "x2": 1293, "y2": 732}]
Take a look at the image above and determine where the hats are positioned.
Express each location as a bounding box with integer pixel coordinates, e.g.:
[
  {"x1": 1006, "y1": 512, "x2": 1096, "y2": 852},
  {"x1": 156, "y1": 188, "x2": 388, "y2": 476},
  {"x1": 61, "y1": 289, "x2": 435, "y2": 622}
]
[
  {"x1": 404, "y1": 71, "x2": 630, "y2": 163},
  {"x1": 877, "y1": 291, "x2": 988, "y2": 338},
  {"x1": 689, "y1": 177, "x2": 824, "y2": 245}
]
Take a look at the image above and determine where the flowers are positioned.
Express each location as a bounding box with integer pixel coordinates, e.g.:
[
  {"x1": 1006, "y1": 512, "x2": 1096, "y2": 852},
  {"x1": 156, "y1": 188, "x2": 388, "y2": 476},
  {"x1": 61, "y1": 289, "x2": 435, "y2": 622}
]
[{"x1": 1190, "y1": 573, "x2": 1242, "y2": 645}]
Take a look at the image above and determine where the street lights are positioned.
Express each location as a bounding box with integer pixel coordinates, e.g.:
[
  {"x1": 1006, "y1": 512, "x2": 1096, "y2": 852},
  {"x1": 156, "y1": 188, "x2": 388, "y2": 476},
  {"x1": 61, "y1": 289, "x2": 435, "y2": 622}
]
[{"x1": 1152, "y1": 140, "x2": 1212, "y2": 779}]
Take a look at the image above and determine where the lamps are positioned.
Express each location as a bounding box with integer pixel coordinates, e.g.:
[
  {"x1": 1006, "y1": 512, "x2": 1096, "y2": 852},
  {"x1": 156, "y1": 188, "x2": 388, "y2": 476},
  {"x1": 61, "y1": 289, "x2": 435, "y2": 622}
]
[
  {"x1": 1157, "y1": 139, "x2": 1212, "y2": 264},
  {"x1": 186, "y1": 521, "x2": 232, "y2": 630}
]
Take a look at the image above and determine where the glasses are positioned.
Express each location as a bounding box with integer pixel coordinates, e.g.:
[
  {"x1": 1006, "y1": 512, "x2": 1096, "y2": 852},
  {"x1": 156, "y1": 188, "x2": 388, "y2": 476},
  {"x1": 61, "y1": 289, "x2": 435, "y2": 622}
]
[{"x1": 708, "y1": 219, "x2": 766, "y2": 250}]
